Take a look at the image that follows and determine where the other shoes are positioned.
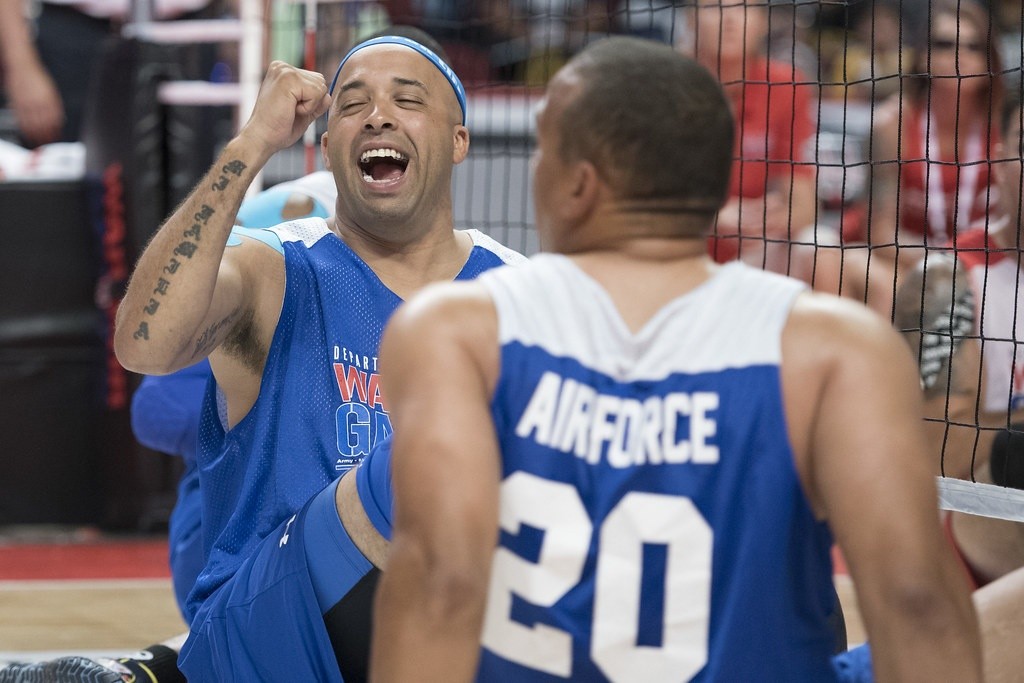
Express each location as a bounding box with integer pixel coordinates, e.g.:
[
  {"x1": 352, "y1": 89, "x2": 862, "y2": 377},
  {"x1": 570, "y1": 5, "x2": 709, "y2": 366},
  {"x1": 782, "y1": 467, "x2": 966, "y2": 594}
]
[{"x1": 0, "y1": 648, "x2": 154, "y2": 683}]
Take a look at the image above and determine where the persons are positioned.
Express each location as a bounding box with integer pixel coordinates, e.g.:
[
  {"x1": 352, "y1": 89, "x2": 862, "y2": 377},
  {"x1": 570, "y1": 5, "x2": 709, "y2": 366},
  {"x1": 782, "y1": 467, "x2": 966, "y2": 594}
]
[
  {"x1": 893, "y1": 91, "x2": 1024, "y2": 586},
  {"x1": 264, "y1": 0, "x2": 1024, "y2": 321},
  {"x1": 113, "y1": 26, "x2": 528, "y2": 683},
  {"x1": 370, "y1": 39, "x2": 1024, "y2": 683},
  {"x1": 132, "y1": 168, "x2": 338, "y2": 683},
  {"x1": 1, "y1": 0, "x2": 239, "y2": 541}
]
